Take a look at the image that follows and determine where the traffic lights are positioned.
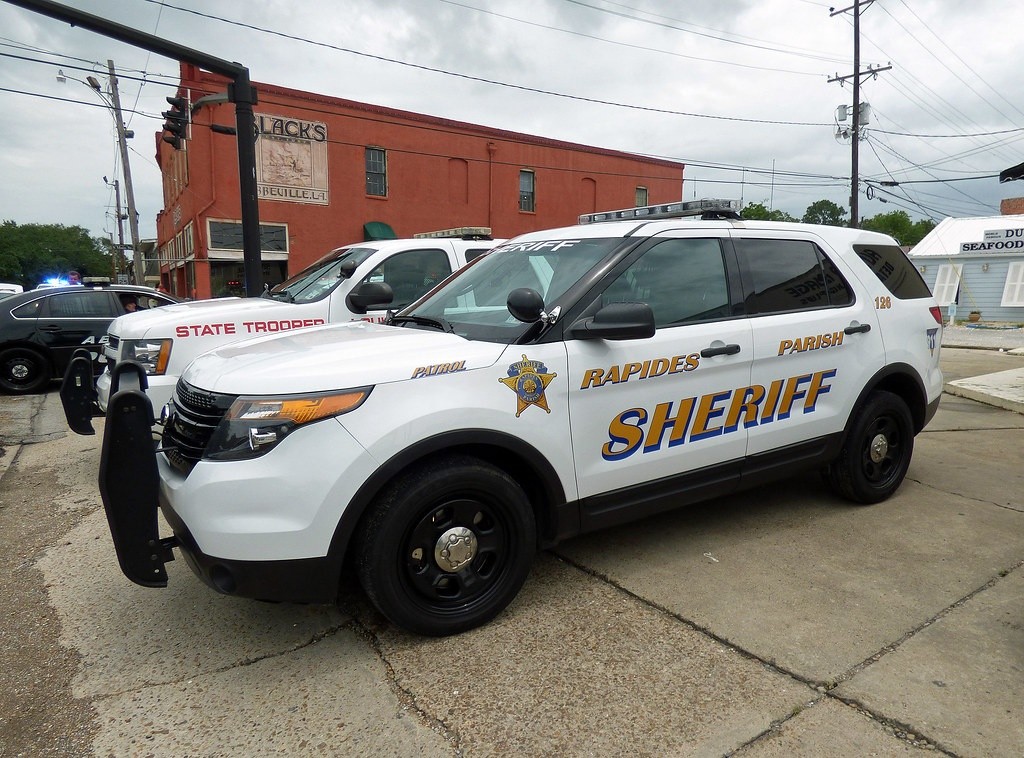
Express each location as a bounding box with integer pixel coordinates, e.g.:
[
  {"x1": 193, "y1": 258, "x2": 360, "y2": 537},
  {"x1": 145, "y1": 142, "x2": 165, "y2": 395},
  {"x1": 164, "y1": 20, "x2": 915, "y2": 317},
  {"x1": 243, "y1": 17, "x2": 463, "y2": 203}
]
[
  {"x1": 160, "y1": 111, "x2": 181, "y2": 150},
  {"x1": 165, "y1": 94, "x2": 187, "y2": 140}
]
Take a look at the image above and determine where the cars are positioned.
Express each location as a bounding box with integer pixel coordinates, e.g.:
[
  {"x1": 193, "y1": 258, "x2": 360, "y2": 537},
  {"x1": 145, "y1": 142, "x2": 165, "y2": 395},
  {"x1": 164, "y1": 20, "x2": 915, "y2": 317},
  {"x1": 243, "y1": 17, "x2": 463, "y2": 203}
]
[
  {"x1": 0, "y1": 279, "x2": 69, "y2": 304},
  {"x1": 0, "y1": 276, "x2": 188, "y2": 397}
]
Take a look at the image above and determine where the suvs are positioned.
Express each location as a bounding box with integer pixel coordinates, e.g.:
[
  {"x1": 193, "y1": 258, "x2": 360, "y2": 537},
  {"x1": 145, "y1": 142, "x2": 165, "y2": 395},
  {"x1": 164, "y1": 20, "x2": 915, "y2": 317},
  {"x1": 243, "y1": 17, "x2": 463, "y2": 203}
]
[
  {"x1": 98, "y1": 199, "x2": 948, "y2": 639},
  {"x1": 60, "y1": 227, "x2": 564, "y2": 452}
]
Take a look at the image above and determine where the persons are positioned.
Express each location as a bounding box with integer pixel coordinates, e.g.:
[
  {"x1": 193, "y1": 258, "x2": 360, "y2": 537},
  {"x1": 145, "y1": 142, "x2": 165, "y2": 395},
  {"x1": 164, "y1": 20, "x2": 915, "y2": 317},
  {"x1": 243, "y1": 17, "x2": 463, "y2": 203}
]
[
  {"x1": 157, "y1": 284, "x2": 167, "y2": 292},
  {"x1": 69, "y1": 270, "x2": 81, "y2": 285},
  {"x1": 118, "y1": 293, "x2": 137, "y2": 313}
]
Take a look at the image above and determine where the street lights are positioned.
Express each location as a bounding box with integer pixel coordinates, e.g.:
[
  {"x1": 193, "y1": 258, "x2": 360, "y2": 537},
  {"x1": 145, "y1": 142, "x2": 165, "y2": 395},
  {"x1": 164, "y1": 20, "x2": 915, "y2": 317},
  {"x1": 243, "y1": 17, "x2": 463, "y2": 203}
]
[{"x1": 56, "y1": 57, "x2": 145, "y2": 286}]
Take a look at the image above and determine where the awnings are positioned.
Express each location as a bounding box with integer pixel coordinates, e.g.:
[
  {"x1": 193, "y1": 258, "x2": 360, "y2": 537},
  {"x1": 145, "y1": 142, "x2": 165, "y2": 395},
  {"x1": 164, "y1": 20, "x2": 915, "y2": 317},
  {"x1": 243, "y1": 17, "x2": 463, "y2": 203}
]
[{"x1": 364, "y1": 222, "x2": 398, "y2": 239}]
